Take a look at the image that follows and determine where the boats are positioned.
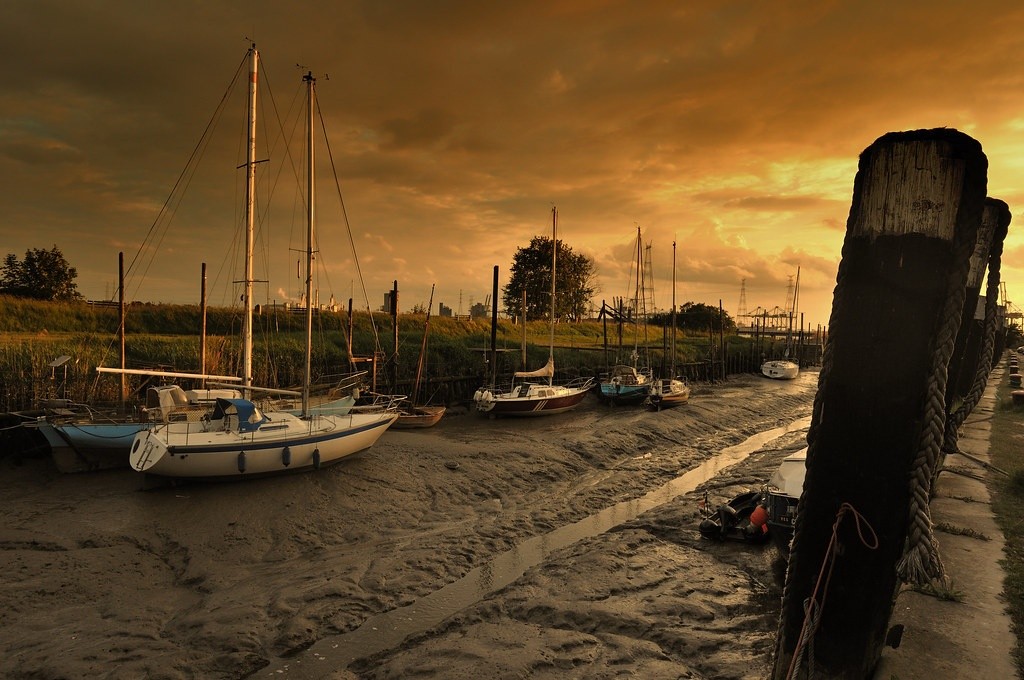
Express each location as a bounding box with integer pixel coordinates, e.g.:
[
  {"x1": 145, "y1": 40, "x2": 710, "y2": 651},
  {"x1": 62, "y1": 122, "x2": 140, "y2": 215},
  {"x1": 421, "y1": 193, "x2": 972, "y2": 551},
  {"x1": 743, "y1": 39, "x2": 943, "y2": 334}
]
[
  {"x1": 768, "y1": 444, "x2": 811, "y2": 563},
  {"x1": 700, "y1": 486, "x2": 771, "y2": 543}
]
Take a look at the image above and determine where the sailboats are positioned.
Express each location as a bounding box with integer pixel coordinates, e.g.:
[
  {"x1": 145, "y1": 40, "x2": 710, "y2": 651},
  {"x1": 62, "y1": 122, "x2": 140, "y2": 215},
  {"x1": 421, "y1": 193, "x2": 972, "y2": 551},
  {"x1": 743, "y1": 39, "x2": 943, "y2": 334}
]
[{"x1": 1, "y1": 45, "x2": 805, "y2": 478}]
